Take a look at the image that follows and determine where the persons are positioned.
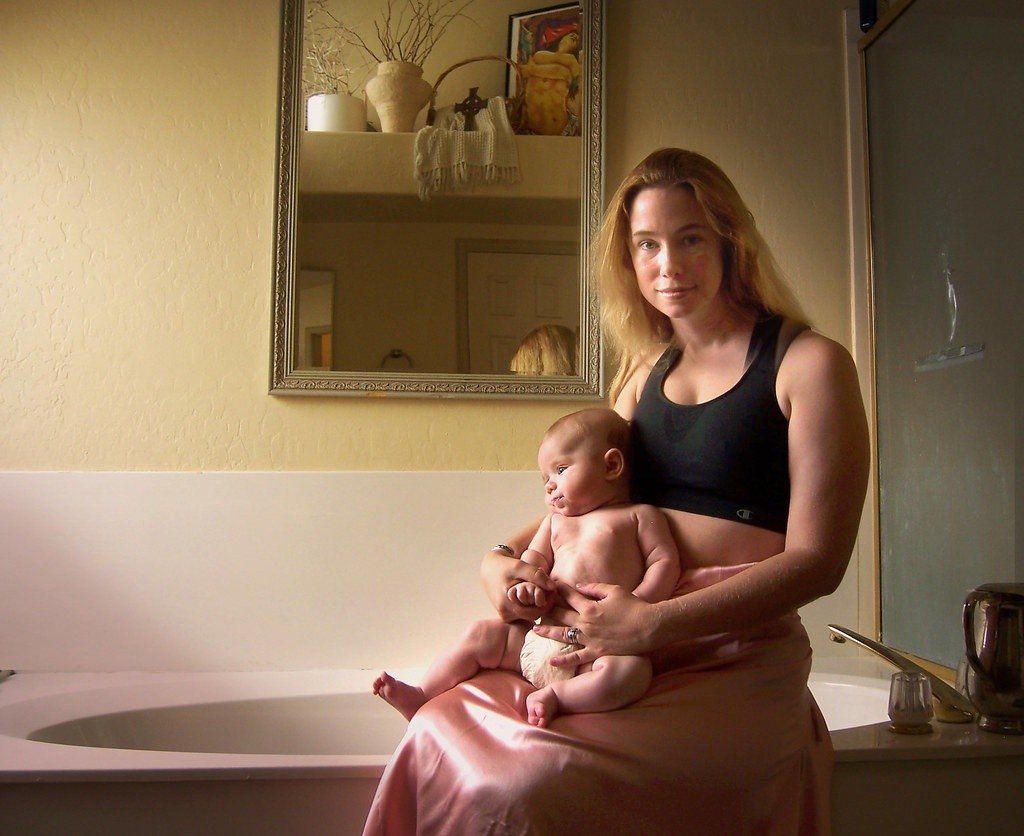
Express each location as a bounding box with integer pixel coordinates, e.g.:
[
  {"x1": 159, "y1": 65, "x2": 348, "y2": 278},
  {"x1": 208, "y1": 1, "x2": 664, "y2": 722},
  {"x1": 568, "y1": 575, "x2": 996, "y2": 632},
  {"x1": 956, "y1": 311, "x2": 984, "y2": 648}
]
[
  {"x1": 511, "y1": 325, "x2": 575, "y2": 378},
  {"x1": 373, "y1": 409, "x2": 680, "y2": 727},
  {"x1": 360, "y1": 149, "x2": 871, "y2": 836}
]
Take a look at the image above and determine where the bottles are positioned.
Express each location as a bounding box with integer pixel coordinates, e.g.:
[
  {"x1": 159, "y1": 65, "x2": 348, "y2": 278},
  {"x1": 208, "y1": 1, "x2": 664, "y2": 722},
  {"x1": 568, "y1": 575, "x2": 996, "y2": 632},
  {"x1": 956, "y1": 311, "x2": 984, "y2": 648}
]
[
  {"x1": 961, "y1": 582, "x2": 1024, "y2": 732},
  {"x1": 888, "y1": 670, "x2": 933, "y2": 729}
]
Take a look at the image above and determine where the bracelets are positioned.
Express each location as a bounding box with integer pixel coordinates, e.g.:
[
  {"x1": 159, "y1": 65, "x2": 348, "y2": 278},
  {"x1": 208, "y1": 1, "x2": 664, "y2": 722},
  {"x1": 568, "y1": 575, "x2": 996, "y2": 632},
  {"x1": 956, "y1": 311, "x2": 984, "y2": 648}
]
[{"x1": 491, "y1": 544, "x2": 514, "y2": 555}]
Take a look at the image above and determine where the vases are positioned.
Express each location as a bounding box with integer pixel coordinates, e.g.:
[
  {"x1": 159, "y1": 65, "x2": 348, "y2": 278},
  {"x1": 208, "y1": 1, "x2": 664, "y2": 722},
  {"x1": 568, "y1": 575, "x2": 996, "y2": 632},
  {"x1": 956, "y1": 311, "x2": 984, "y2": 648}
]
[
  {"x1": 365, "y1": 62, "x2": 434, "y2": 132},
  {"x1": 305, "y1": 91, "x2": 367, "y2": 133}
]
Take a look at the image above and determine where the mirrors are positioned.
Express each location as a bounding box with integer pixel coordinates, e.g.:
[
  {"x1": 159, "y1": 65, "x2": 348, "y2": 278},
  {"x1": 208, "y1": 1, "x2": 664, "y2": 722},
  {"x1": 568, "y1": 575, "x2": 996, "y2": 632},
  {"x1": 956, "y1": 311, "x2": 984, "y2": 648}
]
[{"x1": 268, "y1": 0, "x2": 607, "y2": 403}]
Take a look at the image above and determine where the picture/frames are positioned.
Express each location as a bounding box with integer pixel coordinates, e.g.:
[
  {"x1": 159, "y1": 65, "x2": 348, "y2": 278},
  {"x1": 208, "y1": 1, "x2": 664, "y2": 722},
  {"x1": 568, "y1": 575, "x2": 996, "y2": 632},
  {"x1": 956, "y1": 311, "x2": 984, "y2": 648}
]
[{"x1": 504, "y1": 1, "x2": 583, "y2": 138}]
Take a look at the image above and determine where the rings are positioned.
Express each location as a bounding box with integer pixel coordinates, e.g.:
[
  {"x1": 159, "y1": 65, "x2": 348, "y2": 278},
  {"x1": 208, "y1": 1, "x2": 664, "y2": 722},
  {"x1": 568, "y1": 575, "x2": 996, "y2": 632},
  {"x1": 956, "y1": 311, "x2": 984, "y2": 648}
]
[{"x1": 569, "y1": 628, "x2": 578, "y2": 644}]
